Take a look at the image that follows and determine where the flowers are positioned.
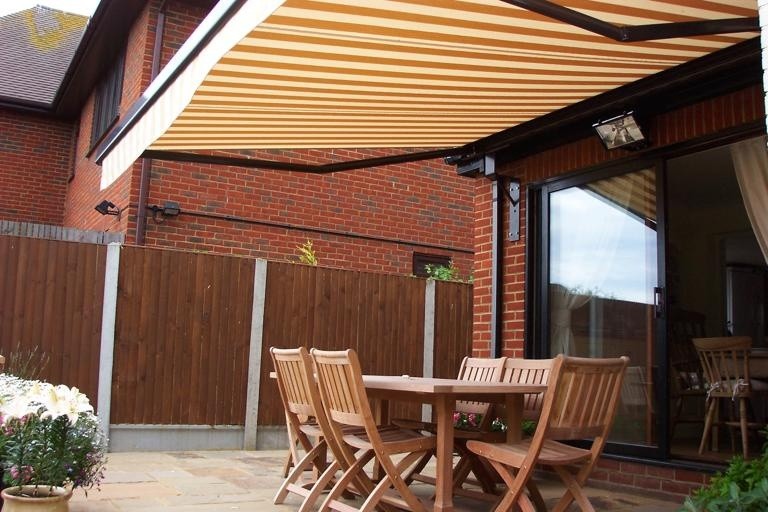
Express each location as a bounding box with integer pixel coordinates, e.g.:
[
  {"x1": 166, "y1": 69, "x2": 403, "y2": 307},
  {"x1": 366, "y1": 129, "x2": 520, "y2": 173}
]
[{"x1": 0, "y1": 339, "x2": 112, "y2": 499}]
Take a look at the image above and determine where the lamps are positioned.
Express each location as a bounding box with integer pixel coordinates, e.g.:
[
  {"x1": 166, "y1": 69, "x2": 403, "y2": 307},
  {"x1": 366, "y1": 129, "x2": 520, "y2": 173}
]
[
  {"x1": 96, "y1": 199, "x2": 121, "y2": 219},
  {"x1": 592, "y1": 111, "x2": 648, "y2": 153},
  {"x1": 151, "y1": 201, "x2": 180, "y2": 224}
]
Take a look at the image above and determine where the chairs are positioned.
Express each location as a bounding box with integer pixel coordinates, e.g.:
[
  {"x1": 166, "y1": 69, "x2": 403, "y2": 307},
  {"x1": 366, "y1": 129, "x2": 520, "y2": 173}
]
[{"x1": 691, "y1": 334, "x2": 762, "y2": 461}]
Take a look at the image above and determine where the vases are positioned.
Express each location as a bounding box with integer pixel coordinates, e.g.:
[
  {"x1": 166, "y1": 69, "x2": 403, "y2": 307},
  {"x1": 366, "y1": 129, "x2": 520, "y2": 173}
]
[{"x1": 0, "y1": 484, "x2": 73, "y2": 512}]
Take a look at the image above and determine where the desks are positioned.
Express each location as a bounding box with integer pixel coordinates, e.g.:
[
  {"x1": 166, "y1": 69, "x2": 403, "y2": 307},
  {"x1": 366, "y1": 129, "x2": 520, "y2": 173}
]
[{"x1": 696, "y1": 347, "x2": 768, "y2": 453}]
